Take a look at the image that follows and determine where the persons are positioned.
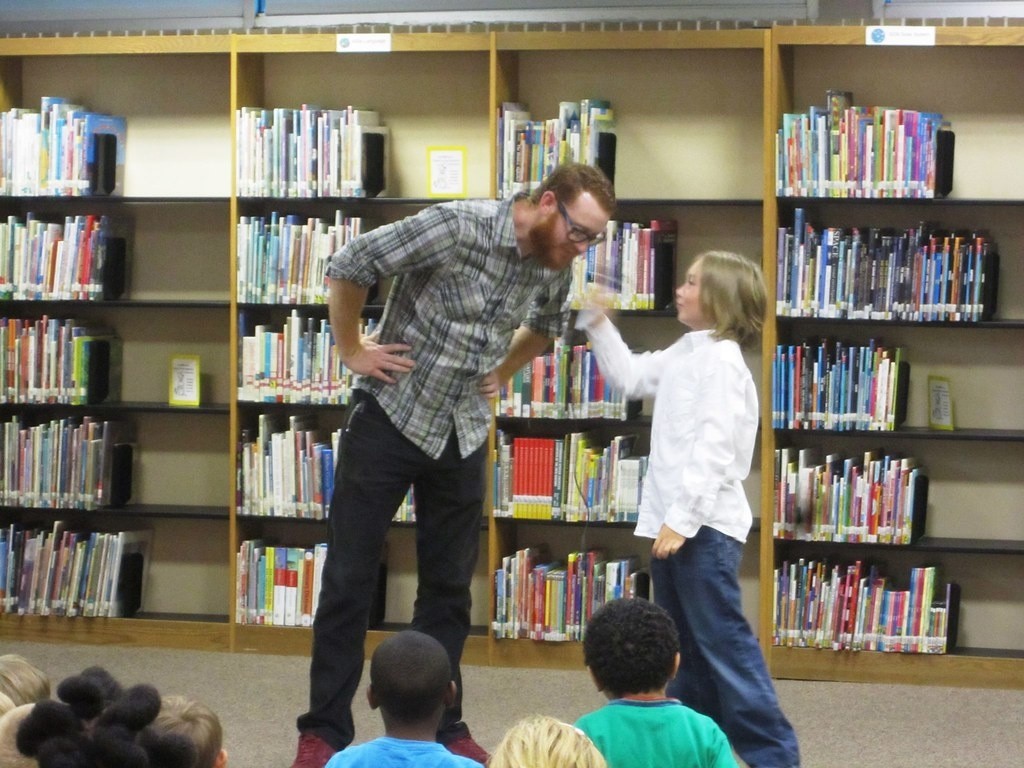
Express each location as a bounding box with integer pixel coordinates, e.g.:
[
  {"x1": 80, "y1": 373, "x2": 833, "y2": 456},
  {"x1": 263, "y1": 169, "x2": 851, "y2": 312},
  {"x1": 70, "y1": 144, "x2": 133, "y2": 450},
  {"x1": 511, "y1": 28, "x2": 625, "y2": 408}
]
[
  {"x1": 292, "y1": 164, "x2": 619, "y2": 768},
  {"x1": 572, "y1": 597, "x2": 739, "y2": 767},
  {"x1": 322, "y1": 631, "x2": 485, "y2": 767},
  {"x1": 1, "y1": 654, "x2": 607, "y2": 768},
  {"x1": 576, "y1": 248, "x2": 801, "y2": 768}
]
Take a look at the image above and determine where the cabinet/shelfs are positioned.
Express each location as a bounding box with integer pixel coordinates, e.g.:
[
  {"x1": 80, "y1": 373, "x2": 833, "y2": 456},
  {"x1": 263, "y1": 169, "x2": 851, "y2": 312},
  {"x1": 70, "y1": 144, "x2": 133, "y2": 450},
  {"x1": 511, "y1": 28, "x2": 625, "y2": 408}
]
[
  {"x1": 0, "y1": 18, "x2": 772, "y2": 674},
  {"x1": 767, "y1": 15, "x2": 1024, "y2": 690}
]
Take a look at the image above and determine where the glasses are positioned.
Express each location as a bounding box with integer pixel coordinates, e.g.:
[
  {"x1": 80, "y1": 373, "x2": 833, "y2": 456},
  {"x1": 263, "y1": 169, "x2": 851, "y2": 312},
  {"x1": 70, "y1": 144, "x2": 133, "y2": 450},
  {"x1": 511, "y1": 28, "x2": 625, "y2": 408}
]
[{"x1": 556, "y1": 197, "x2": 606, "y2": 246}]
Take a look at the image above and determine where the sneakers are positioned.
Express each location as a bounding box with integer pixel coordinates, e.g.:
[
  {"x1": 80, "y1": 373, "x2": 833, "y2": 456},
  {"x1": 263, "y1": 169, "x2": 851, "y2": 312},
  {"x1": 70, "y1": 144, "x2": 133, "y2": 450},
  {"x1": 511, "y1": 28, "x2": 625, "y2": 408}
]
[
  {"x1": 446, "y1": 734, "x2": 489, "y2": 763},
  {"x1": 291, "y1": 732, "x2": 336, "y2": 768}
]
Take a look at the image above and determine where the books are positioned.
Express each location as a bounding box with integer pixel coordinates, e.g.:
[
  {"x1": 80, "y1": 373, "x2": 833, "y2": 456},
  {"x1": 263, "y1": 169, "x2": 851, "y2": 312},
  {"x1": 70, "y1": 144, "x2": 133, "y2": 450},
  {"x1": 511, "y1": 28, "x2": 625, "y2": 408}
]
[
  {"x1": 1, "y1": 414, "x2": 139, "y2": 511},
  {"x1": 236, "y1": 207, "x2": 416, "y2": 628},
  {"x1": 0, "y1": 316, "x2": 122, "y2": 405},
  {"x1": 495, "y1": 210, "x2": 679, "y2": 646},
  {"x1": 1, "y1": 96, "x2": 126, "y2": 197},
  {"x1": 496, "y1": 98, "x2": 615, "y2": 200},
  {"x1": 0, "y1": 522, "x2": 154, "y2": 618},
  {"x1": 235, "y1": 105, "x2": 392, "y2": 199},
  {"x1": 775, "y1": 89, "x2": 999, "y2": 653},
  {"x1": 0, "y1": 215, "x2": 134, "y2": 301}
]
